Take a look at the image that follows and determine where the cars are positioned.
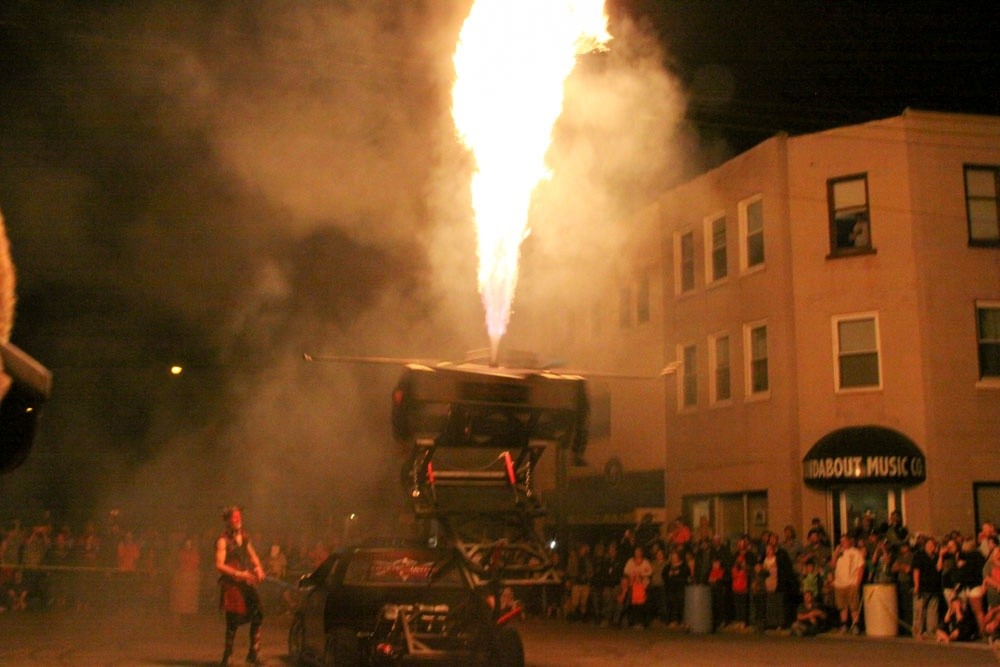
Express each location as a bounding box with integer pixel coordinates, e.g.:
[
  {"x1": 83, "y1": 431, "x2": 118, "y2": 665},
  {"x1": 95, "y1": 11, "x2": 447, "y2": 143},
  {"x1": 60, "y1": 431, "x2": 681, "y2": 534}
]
[{"x1": 288, "y1": 541, "x2": 526, "y2": 667}]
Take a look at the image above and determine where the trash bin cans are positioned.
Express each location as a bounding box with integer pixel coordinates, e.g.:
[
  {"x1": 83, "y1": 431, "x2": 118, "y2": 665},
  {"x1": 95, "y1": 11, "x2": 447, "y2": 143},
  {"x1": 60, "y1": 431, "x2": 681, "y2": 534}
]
[
  {"x1": 863, "y1": 583, "x2": 898, "y2": 637},
  {"x1": 684, "y1": 584, "x2": 712, "y2": 634}
]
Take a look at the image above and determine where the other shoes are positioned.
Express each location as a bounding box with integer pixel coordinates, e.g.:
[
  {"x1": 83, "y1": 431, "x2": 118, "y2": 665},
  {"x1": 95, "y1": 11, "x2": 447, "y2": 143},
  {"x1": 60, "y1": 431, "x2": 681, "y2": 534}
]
[
  {"x1": 546, "y1": 606, "x2": 999, "y2": 642},
  {"x1": 247, "y1": 654, "x2": 269, "y2": 667}
]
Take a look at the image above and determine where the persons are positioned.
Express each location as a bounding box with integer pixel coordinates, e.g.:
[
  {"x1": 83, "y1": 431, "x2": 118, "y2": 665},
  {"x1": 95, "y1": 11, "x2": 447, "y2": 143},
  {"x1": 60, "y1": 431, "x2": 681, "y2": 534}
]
[
  {"x1": 0, "y1": 506, "x2": 338, "y2": 626},
  {"x1": 214, "y1": 505, "x2": 264, "y2": 667},
  {"x1": 469, "y1": 510, "x2": 999, "y2": 646}
]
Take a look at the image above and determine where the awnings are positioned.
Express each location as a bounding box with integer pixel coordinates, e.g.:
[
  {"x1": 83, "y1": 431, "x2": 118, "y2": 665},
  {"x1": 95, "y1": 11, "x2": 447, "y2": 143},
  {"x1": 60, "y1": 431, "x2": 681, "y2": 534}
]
[{"x1": 802, "y1": 424, "x2": 927, "y2": 488}]
